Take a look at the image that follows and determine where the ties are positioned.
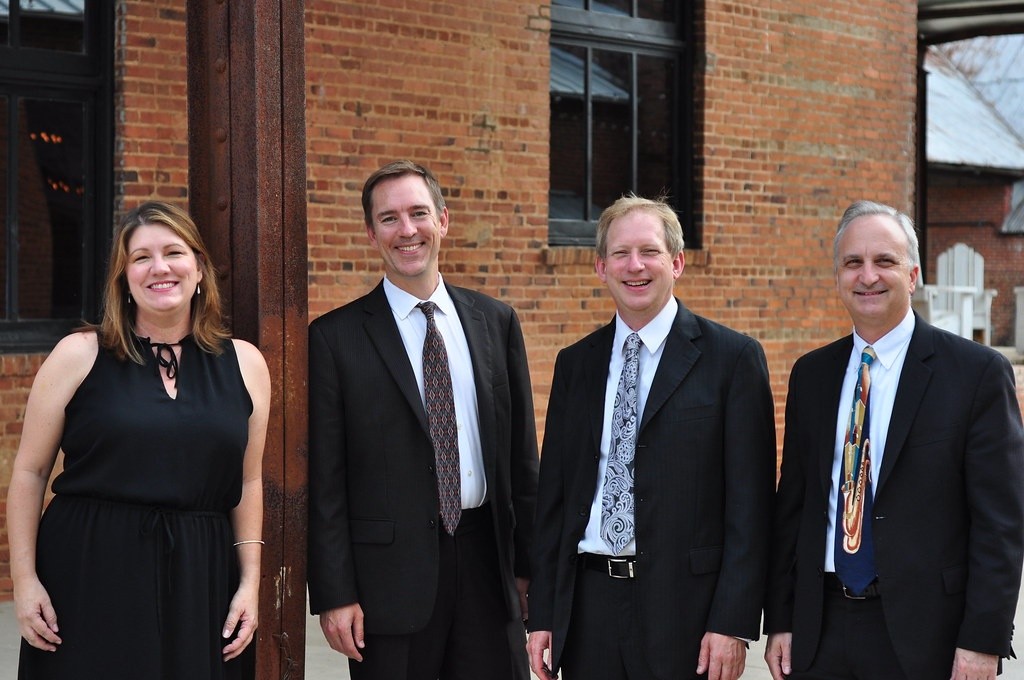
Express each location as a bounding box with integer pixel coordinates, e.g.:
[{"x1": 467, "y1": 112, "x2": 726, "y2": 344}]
[
  {"x1": 834, "y1": 346, "x2": 878, "y2": 596},
  {"x1": 415, "y1": 301, "x2": 462, "y2": 536},
  {"x1": 600, "y1": 332, "x2": 643, "y2": 556}
]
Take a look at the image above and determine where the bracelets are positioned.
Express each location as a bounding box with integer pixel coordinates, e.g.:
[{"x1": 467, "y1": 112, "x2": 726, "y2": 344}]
[{"x1": 233, "y1": 540, "x2": 265, "y2": 546}]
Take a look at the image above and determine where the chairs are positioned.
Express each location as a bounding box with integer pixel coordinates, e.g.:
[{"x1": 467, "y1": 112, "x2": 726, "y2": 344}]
[
  {"x1": 913, "y1": 246, "x2": 975, "y2": 341},
  {"x1": 936, "y1": 244, "x2": 998, "y2": 347}
]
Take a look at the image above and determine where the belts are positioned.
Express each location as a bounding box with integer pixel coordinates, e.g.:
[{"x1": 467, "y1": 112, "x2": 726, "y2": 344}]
[
  {"x1": 826, "y1": 580, "x2": 879, "y2": 600},
  {"x1": 579, "y1": 553, "x2": 637, "y2": 580}
]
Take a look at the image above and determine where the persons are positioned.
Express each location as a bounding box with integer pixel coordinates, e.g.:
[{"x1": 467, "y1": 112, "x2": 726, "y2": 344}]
[
  {"x1": 763, "y1": 201, "x2": 1024, "y2": 680},
  {"x1": 308, "y1": 161, "x2": 540, "y2": 680},
  {"x1": 6, "y1": 199, "x2": 271, "y2": 680},
  {"x1": 524, "y1": 185, "x2": 777, "y2": 680}
]
